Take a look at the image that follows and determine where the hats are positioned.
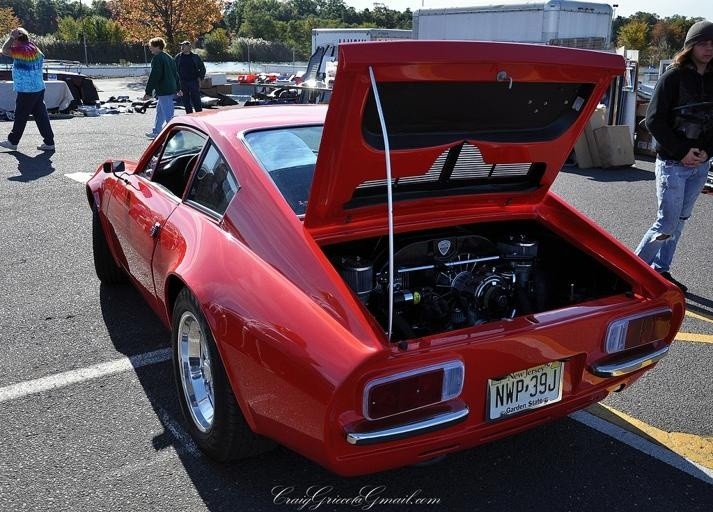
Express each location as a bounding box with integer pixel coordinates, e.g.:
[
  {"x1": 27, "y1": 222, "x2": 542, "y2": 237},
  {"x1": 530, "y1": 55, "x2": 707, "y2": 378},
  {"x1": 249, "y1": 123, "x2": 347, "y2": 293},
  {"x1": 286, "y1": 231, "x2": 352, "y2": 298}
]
[
  {"x1": 684, "y1": 21, "x2": 713, "y2": 47},
  {"x1": 179, "y1": 41, "x2": 191, "y2": 48}
]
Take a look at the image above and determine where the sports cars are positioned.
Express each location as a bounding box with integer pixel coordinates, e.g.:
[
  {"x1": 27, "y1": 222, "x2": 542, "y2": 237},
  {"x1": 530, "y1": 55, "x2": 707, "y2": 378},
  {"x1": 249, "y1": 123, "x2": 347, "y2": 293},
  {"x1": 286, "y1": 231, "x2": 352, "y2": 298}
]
[{"x1": 78, "y1": 38, "x2": 687, "y2": 483}]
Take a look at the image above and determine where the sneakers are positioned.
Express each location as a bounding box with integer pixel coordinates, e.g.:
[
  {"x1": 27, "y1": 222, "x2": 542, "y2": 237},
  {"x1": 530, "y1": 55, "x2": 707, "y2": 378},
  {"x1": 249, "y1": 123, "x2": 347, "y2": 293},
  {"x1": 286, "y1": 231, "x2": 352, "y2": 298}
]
[
  {"x1": 38, "y1": 143, "x2": 55, "y2": 150},
  {"x1": 661, "y1": 272, "x2": 688, "y2": 292},
  {"x1": 146, "y1": 132, "x2": 158, "y2": 138},
  {"x1": 0, "y1": 141, "x2": 17, "y2": 150}
]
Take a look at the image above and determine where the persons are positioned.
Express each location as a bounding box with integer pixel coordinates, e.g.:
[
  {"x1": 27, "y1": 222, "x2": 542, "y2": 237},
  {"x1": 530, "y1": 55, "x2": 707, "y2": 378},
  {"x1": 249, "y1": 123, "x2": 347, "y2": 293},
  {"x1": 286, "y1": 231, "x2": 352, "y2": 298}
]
[
  {"x1": 174, "y1": 41, "x2": 206, "y2": 114},
  {"x1": 0, "y1": 26, "x2": 55, "y2": 150},
  {"x1": 142, "y1": 37, "x2": 182, "y2": 139},
  {"x1": 636, "y1": 20, "x2": 713, "y2": 292}
]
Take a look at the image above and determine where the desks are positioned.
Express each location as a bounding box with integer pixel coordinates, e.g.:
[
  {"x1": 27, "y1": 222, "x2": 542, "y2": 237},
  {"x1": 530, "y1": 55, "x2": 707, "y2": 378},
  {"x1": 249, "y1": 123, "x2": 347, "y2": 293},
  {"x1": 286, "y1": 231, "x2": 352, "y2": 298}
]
[{"x1": 236, "y1": 82, "x2": 333, "y2": 104}]
